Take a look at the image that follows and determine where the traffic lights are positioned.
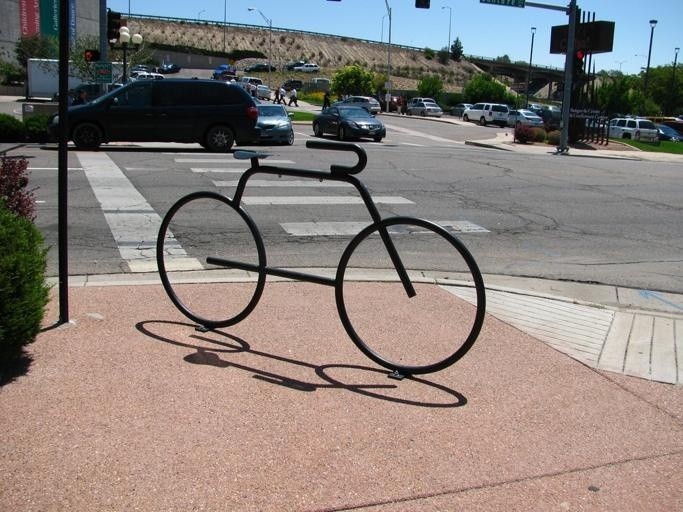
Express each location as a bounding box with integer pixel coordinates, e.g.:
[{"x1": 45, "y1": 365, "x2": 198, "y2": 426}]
[
  {"x1": 84, "y1": 49, "x2": 98, "y2": 60},
  {"x1": 107, "y1": 10, "x2": 119, "y2": 40},
  {"x1": 571, "y1": 49, "x2": 587, "y2": 78}
]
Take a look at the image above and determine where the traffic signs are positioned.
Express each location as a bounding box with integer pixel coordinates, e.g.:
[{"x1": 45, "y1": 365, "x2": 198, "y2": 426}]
[{"x1": 479, "y1": 0, "x2": 525, "y2": 9}]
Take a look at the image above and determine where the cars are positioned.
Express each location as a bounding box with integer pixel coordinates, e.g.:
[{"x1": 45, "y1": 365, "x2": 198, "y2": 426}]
[
  {"x1": 24, "y1": 59, "x2": 276, "y2": 103},
  {"x1": 238, "y1": 105, "x2": 295, "y2": 143}
]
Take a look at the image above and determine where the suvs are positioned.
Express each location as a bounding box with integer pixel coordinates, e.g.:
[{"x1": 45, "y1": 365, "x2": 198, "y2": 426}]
[
  {"x1": 281, "y1": 80, "x2": 303, "y2": 91},
  {"x1": 283, "y1": 61, "x2": 305, "y2": 71},
  {"x1": 293, "y1": 63, "x2": 320, "y2": 74}
]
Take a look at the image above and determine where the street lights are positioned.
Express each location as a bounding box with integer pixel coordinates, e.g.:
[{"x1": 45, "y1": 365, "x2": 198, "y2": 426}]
[
  {"x1": 642, "y1": 19, "x2": 657, "y2": 114},
  {"x1": 108, "y1": 26, "x2": 143, "y2": 85},
  {"x1": 525, "y1": 26, "x2": 537, "y2": 108},
  {"x1": 663, "y1": 48, "x2": 680, "y2": 115},
  {"x1": 248, "y1": 7, "x2": 271, "y2": 91},
  {"x1": 440, "y1": 7, "x2": 451, "y2": 54}
]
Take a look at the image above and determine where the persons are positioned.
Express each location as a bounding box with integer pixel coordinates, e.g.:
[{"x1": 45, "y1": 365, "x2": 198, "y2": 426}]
[
  {"x1": 288, "y1": 86, "x2": 299, "y2": 107},
  {"x1": 278, "y1": 85, "x2": 287, "y2": 105},
  {"x1": 68, "y1": 89, "x2": 89, "y2": 106},
  {"x1": 273, "y1": 88, "x2": 280, "y2": 104},
  {"x1": 322, "y1": 91, "x2": 408, "y2": 115},
  {"x1": 210, "y1": 76, "x2": 257, "y2": 97}
]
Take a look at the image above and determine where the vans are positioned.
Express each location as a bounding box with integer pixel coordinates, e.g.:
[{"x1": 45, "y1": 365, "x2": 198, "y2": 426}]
[
  {"x1": 312, "y1": 78, "x2": 332, "y2": 89},
  {"x1": 45, "y1": 79, "x2": 259, "y2": 153}
]
